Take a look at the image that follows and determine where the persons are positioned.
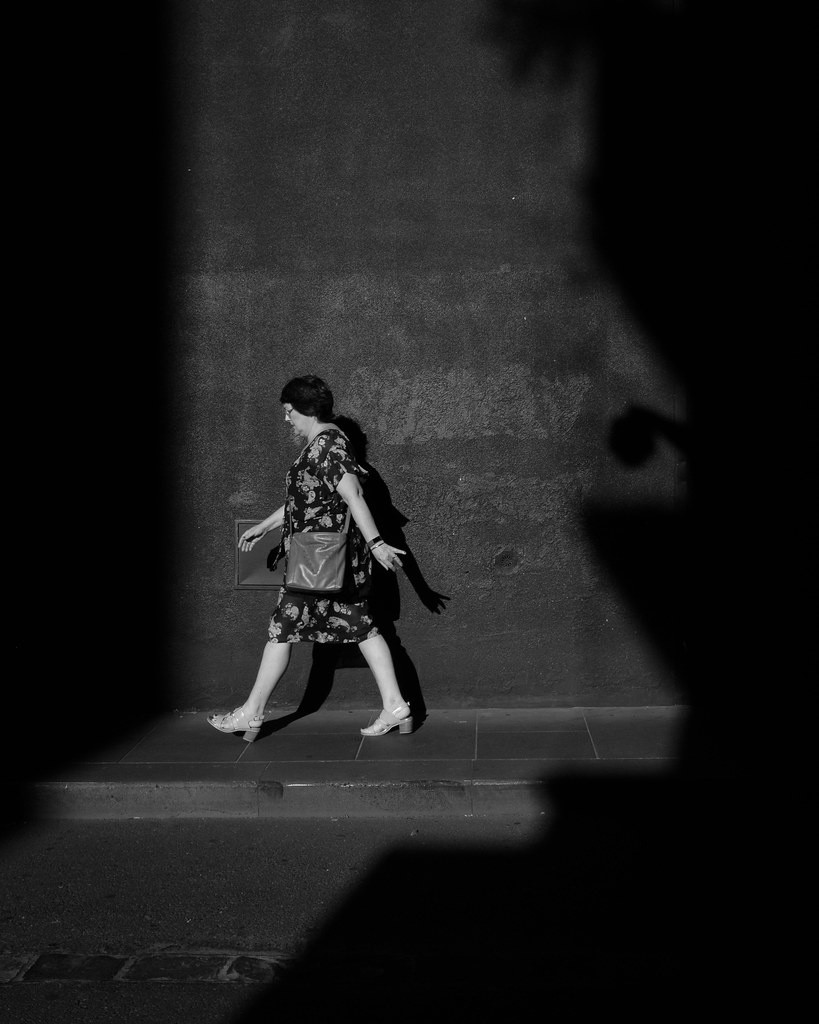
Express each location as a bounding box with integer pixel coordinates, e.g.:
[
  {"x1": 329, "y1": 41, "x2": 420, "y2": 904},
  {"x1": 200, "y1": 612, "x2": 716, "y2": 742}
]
[{"x1": 205, "y1": 375, "x2": 414, "y2": 742}]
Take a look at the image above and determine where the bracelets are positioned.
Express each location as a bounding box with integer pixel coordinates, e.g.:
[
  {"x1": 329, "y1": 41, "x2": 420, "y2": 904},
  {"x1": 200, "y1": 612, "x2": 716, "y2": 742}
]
[
  {"x1": 367, "y1": 536, "x2": 384, "y2": 547},
  {"x1": 370, "y1": 542, "x2": 386, "y2": 552}
]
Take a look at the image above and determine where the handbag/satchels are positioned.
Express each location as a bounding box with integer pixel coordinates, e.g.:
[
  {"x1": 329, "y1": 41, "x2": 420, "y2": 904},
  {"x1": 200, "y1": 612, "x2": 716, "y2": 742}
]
[{"x1": 285, "y1": 532, "x2": 347, "y2": 592}]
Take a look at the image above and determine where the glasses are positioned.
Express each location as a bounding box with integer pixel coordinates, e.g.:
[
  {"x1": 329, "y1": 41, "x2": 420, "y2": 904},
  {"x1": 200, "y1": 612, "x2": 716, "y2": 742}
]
[{"x1": 285, "y1": 407, "x2": 293, "y2": 415}]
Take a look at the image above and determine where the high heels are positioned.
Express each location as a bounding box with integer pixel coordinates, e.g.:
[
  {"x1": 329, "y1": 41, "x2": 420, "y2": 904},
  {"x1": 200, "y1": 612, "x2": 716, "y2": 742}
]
[
  {"x1": 361, "y1": 702, "x2": 412, "y2": 736},
  {"x1": 208, "y1": 705, "x2": 266, "y2": 742}
]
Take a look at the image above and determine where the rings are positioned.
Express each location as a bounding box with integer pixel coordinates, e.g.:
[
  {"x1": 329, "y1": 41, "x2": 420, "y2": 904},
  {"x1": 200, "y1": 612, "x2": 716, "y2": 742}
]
[{"x1": 392, "y1": 554, "x2": 399, "y2": 559}]
